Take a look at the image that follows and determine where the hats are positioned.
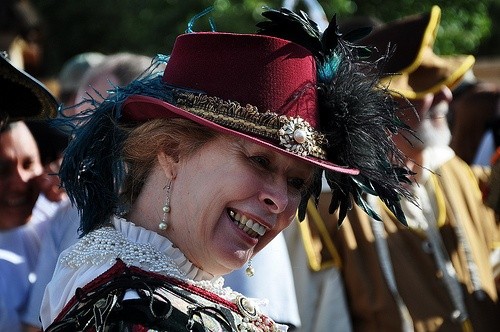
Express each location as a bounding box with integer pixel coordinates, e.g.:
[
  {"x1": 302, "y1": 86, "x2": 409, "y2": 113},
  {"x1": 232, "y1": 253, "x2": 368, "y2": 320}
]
[
  {"x1": 0, "y1": 54, "x2": 60, "y2": 122},
  {"x1": 122, "y1": 33, "x2": 360, "y2": 176},
  {"x1": 353, "y1": 6, "x2": 475, "y2": 100}
]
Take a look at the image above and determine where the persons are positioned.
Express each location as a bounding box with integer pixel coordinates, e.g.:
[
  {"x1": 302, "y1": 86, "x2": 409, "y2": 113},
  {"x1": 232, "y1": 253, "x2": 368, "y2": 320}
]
[
  {"x1": 39, "y1": 7, "x2": 417, "y2": 332},
  {"x1": 281, "y1": 4, "x2": 499, "y2": 332},
  {"x1": 0, "y1": 51, "x2": 301, "y2": 332}
]
